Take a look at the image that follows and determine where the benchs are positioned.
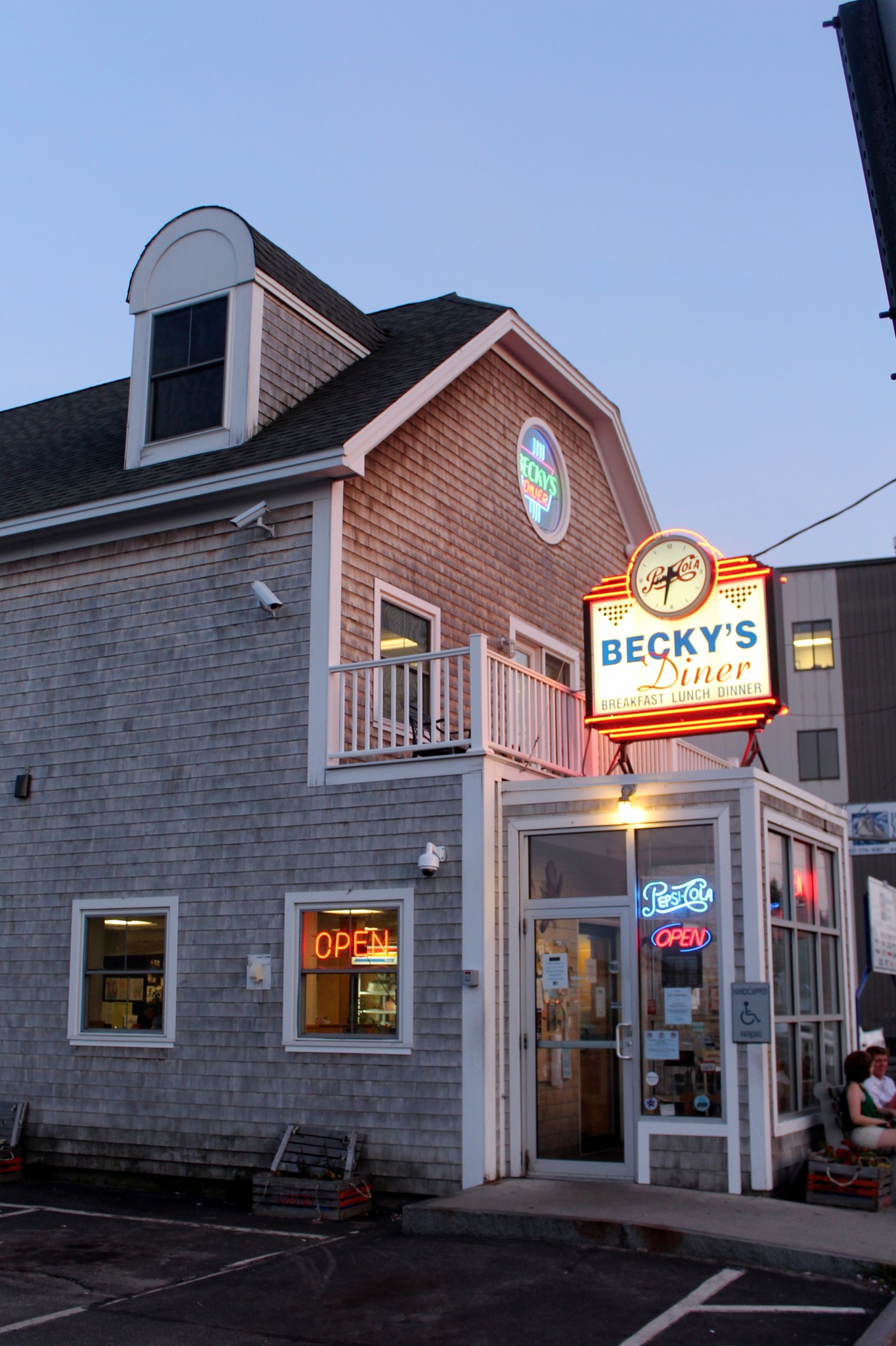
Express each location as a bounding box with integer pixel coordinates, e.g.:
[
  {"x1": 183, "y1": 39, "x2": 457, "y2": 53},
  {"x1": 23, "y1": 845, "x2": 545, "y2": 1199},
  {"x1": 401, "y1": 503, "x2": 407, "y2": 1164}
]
[{"x1": 812, "y1": 1082, "x2": 896, "y2": 1201}]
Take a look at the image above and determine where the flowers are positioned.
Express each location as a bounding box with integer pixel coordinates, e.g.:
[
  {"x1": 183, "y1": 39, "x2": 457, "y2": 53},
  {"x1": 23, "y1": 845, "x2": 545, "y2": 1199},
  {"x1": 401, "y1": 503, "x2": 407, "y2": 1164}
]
[
  {"x1": 0, "y1": 1139, "x2": 5, "y2": 1144},
  {"x1": 296, "y1": 1139, "x2": 343, "y2": 1181},
  {"x1": 821, "y1": 1138, "x2": 891, "y2": 1169}
]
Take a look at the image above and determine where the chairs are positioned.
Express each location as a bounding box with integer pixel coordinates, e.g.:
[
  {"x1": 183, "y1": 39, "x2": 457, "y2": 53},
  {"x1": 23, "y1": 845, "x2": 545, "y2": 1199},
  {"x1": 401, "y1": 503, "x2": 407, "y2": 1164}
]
[{"x1": 400, "y1": 701, "x2": 472, "y2": 758}]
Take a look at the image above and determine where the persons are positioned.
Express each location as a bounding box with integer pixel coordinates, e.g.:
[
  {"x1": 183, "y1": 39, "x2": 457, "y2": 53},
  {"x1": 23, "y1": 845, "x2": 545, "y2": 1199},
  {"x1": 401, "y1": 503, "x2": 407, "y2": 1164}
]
[
  {"x1": 862, "y1": 1047, "x2": 896, "y2": 1117},
  {"x1": 843, "y1": 1051, "x2": 896, "y2": 1147}
]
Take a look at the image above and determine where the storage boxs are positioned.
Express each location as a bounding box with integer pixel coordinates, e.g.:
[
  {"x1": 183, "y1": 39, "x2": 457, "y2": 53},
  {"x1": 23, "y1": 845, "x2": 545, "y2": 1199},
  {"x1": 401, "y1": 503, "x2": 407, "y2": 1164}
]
[
  {"x1": 807, "y1": 1161, "x2": 892, "y2": 1212},
  {"x1": 0, "y1": 1147, "x2": 22, "y2": 1183},
  {"x1": 253, "y1": 1172, "x2": 372, "y2": 1219}
]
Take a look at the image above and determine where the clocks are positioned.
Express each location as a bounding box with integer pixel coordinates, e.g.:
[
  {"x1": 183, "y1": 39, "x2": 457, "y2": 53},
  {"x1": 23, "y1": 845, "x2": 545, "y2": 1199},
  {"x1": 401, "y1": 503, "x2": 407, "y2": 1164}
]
[{"x1": 631, "y1": 535, "x2": 714, "y2": 619}]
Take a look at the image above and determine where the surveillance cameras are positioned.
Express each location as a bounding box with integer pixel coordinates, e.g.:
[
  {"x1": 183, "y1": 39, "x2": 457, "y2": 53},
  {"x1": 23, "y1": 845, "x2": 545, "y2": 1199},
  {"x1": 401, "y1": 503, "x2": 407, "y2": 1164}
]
[
  {"x1": 229, "y1": 500, "x2": 269, "y2": 529},
  {"x1": 418, "y1": 852, "x2": 440, "y2": 876},
  {"x1": 250, "y1": 581, "x2": 283, "y2": 611}
]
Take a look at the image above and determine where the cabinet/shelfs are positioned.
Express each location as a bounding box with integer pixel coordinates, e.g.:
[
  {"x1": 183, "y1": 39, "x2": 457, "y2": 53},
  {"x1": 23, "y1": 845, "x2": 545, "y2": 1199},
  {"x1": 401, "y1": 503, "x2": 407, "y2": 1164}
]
[{"x1": 357, "y1": 973, "x2": 397, "y2": 1026}]
